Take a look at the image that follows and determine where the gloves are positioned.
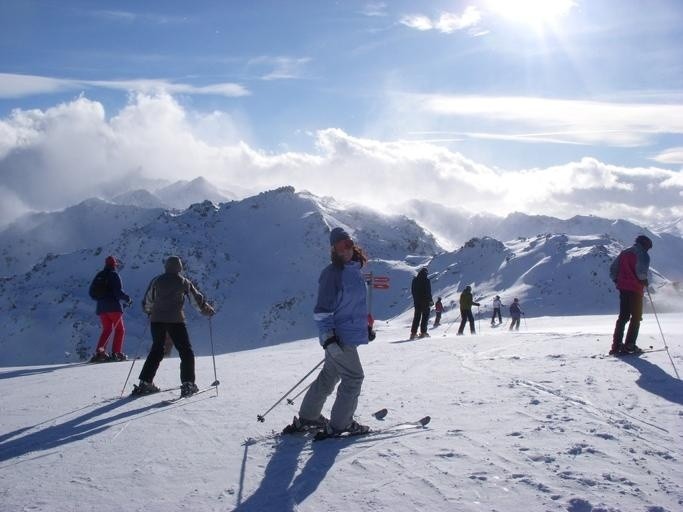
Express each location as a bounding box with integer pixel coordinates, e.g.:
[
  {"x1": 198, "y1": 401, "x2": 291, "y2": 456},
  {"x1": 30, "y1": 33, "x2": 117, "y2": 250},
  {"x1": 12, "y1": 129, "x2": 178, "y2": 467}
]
[{"x1": 324, "y1": 330, "x2": 344, "y2": 358}]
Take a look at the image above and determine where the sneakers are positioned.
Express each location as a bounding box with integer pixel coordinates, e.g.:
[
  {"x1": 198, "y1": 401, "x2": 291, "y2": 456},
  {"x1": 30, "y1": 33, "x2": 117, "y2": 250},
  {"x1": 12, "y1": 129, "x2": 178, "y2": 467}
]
[
  {"x1": 98, "y1": 353, "x2": 110, "y2": 362},
  {"x1": 411, "y1": 332, "x2": 429, "y2": 338},
  {"x1": 181, "y1": 382, "x2": 197, "y2": 395},
  {"x1": 340, "y1": 423, "x2": 369, "y2": 435},
  {"x1": 299, "y1": 412, "x2": 329, "y2": 427},
  {"x1": 139, "y1": 382, "x2": 160, "y2": 393},
  {"x1": 112, "y1": 353, "x2": 127, "y2": 362},
  {"x1": 612, "y1": 343, "x2": 642, "y2": 352}
]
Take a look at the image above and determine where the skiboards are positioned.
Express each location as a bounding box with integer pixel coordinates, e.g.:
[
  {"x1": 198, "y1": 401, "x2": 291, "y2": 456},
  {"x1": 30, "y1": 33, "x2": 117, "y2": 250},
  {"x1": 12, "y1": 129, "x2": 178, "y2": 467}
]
[
  {"x1": 75, "y1": 356, "x2": 139, "y2": 364},
  {"x1": 591, "y1": 347, "x2": 669, "y2": 359},
  {"x1": 390, "y1": 334, "x2": 430, "y2": 343},
  {"x1": 241, "y1": 408, "x2": 430, "y2": 445},
  {"x1": 102, "y1": 382, "x2": 219, "y2": 406}
]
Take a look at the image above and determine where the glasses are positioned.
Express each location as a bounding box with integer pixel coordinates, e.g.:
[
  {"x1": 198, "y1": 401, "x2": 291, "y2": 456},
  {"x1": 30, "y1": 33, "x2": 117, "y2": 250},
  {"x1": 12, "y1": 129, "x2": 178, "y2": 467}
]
[{"x1": 333, "y1": 239, "x2": 353, "y2": 251}]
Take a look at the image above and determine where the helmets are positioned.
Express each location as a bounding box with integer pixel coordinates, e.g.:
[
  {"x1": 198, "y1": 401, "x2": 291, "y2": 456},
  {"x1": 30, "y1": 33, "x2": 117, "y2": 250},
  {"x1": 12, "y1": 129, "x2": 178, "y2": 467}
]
[{"x1": 636, "y1": 235, "x2": 652, "y2": 251}]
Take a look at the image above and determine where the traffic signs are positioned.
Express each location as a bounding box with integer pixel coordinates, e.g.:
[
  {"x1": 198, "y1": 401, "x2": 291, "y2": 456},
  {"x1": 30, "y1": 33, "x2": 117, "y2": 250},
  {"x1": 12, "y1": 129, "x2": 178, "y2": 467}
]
[
  {"x1": 363, "y1": 273, "x2": 372, "y2": 277},
  {"x1": 364, "y1": 278, "x2": 372, "y2": 282},
  {"x1": 373, "y1": 277, "x2": 390, "y2": 282},
  {"x1": 372, "y1": 283, "x2": 390, "y2": 289}
]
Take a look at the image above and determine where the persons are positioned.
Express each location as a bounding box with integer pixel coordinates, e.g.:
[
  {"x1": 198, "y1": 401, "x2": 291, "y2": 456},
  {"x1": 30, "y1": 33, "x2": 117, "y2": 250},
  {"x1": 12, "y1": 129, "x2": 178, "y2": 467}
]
[
  {"x1": 291, "y1": 227, "x2": 379, "y2": 434},
  {"x1": 135, "y1": 256, "x2": 217, "y2": 397},
  {"x1": 455, "y1": 286, "x2": 480, "y2": 335},
  {"x1": 409, "y1": 267, "x2": 433, "y2": 340},
  {"x1": 87, "y1": 254, "x2": 134, "y2": 361},
  {"x1": 434, "y1": 297, "x2": 445, "y2": 325},
  {"x1": 507, "y1": 297, "x2": 525, "y2": 331},
  {"x1": 607, "y1": 234, "x2": 655, "y2": 358},
  {"x1": 490, "y1": 295, "x2": 505, "y2": 324}
]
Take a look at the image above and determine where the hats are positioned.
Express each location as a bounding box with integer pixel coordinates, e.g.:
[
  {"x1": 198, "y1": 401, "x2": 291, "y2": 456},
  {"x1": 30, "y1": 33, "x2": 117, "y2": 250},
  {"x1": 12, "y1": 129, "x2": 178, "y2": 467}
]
[
  {"x1": 331, "y1": 227, "x2": 350, "y2": 244},
  {"x1": 105, "y1": 256, "x2": 118, "y2": 268}
]
